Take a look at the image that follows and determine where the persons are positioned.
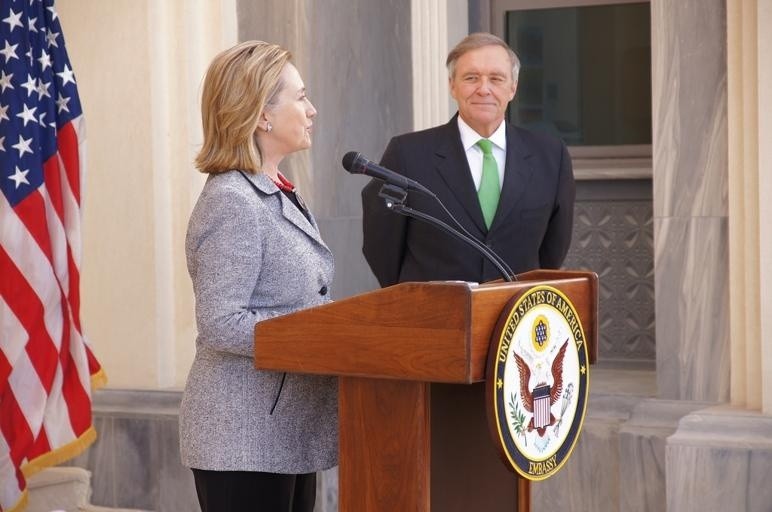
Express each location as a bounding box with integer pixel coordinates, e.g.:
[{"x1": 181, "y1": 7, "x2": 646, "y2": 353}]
[
  {"x1": 176, "y1": 39, "x2": 339, "y2": 512},
  {"x1": 359, "y1": 33, "x2": 577, "y2": 290}
]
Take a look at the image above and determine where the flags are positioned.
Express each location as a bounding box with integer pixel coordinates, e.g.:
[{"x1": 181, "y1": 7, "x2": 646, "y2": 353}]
[{"x1": 0, "y1": 0, "x2": 115, "y2": 511}]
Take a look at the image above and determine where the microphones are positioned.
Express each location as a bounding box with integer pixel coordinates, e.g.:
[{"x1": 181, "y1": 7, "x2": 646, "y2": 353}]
[{"x1": 342, "y1": 151, "x2": 435, "y2": 197}]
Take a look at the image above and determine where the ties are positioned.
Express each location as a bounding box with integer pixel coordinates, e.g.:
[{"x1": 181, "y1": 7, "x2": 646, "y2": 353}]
[{"x1": 477, "y1": 139, "x2": 500, "y2": 230}]
